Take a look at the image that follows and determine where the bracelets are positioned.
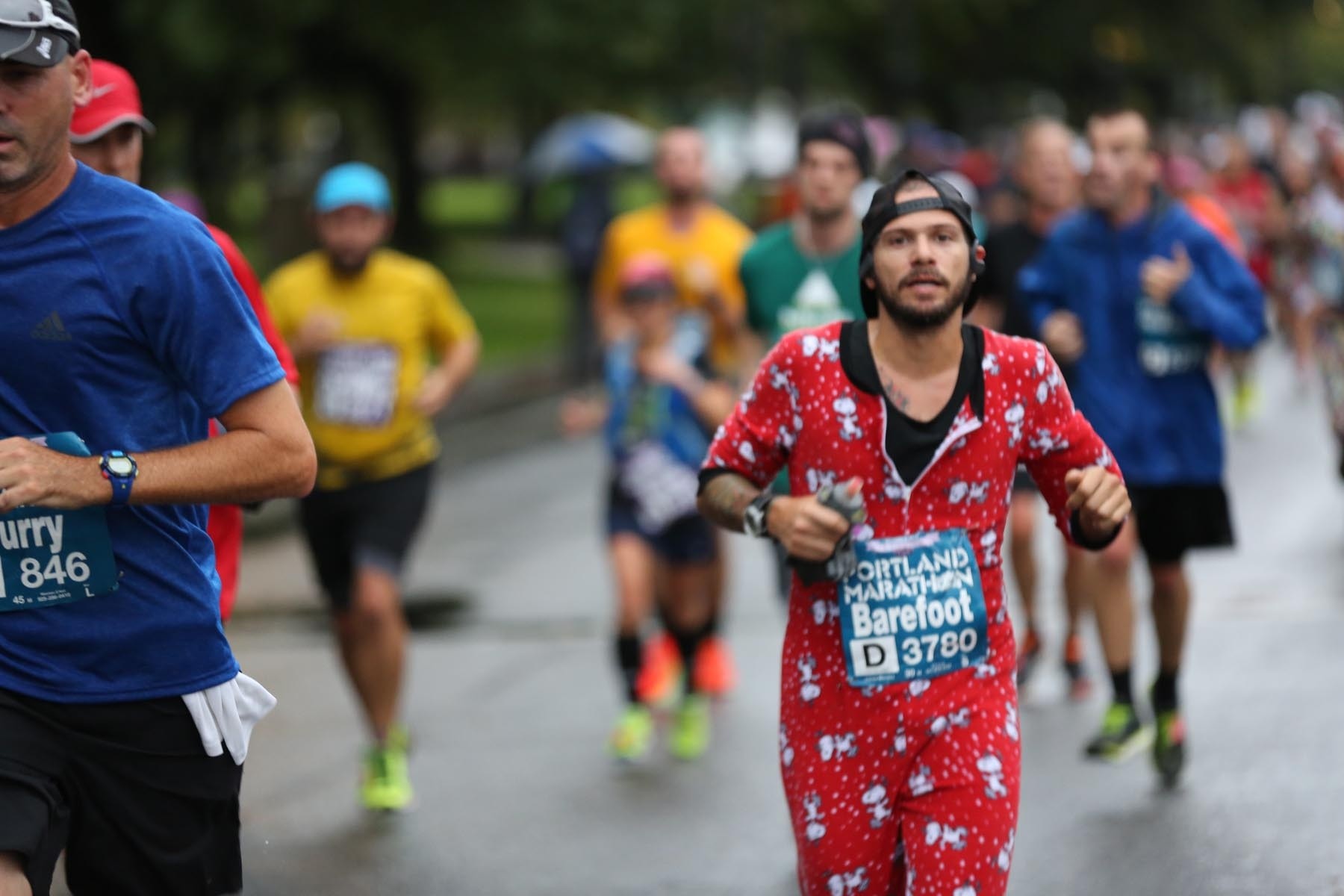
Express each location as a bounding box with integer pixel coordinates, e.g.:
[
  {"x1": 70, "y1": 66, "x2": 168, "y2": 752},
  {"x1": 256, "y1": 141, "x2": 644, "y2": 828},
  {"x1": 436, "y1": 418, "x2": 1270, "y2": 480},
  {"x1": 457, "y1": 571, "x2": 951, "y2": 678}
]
[{"x1": 1066, "y1": 506, "x2": 1128, "y2": 548}]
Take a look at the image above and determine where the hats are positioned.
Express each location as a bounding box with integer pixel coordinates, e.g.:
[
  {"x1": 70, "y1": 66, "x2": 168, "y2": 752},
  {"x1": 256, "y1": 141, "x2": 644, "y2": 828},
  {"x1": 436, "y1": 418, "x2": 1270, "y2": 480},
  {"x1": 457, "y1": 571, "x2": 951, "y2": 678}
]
[
  {"x1": 69, "y1": 59, "x2": 155, "y2": 144},
  {"x1": 615, "y1": 260, "x2": 678, "y2": 304},
  {"x1": 0, "y1": 0, "x2": 80, "y2": 66},
  {"x1": 315, "y1": 161, "x2": 391, "y2": 216},
  {"x1": 858, "y1": 169, "x2": 984, "y2": 320}
]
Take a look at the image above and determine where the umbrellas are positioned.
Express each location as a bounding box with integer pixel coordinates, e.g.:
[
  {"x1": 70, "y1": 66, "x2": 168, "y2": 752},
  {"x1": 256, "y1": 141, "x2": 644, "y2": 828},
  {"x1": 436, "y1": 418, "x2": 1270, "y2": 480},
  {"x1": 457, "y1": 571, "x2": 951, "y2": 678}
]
[{"x1": 525, "y1": 105, "x2": 658, "y2": 190}]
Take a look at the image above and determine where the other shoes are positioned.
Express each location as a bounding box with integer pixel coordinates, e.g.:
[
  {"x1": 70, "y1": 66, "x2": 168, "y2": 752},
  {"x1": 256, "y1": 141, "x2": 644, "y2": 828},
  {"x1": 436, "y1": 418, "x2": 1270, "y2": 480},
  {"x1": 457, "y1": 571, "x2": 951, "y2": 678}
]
[
  {"x1": 1147, "y1": 685, "x2": 1185, "y2": 776},
  {"x1": 611, "y1": 700, "x2": 656, "y2": 765},
  {"x1": 1060, "y1": 635, "x2": 1094, "y2": 704},
  {"x1": 1016, "y1": 629, "x2": 1045, "y2": 688},
  {"x1": 1083, "y1": 702, "x2": 1142, "y2": 757},
  {"x1": 670, "y1": 695, "x2": 711, "y2": 758},
  {"x1": 359, "y1": 725, "x2": 415, "y2": 811}
]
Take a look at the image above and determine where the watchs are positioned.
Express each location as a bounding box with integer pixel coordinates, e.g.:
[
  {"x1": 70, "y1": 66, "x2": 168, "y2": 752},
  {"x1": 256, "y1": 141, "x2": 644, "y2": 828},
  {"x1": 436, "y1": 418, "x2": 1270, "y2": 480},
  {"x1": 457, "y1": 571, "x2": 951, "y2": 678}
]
[
  {"x1": 744, "y1": 490, "x2": 779, "y2": 544},
  {"x1": 98, "y1": 450, "x2": 139, "y2": 509}
]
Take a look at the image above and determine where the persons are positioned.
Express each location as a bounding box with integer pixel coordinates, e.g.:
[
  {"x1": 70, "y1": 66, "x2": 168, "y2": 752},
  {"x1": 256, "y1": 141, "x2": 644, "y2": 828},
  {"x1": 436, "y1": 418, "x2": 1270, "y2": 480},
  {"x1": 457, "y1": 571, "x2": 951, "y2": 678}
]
[
  {"x1": 0, "y1": 0, "x2": 321, "y2": 896},
  {"x1": 70, "y1": 98, "x2": 1344, "y2": 896}
]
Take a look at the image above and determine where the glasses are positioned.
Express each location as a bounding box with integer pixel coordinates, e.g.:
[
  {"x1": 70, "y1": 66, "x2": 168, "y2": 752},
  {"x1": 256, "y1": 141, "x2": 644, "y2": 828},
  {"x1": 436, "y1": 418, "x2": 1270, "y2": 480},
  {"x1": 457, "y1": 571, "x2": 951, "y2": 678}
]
[{"x1": 0, "y1": 0, "x2": 80, "y2": 40}]
